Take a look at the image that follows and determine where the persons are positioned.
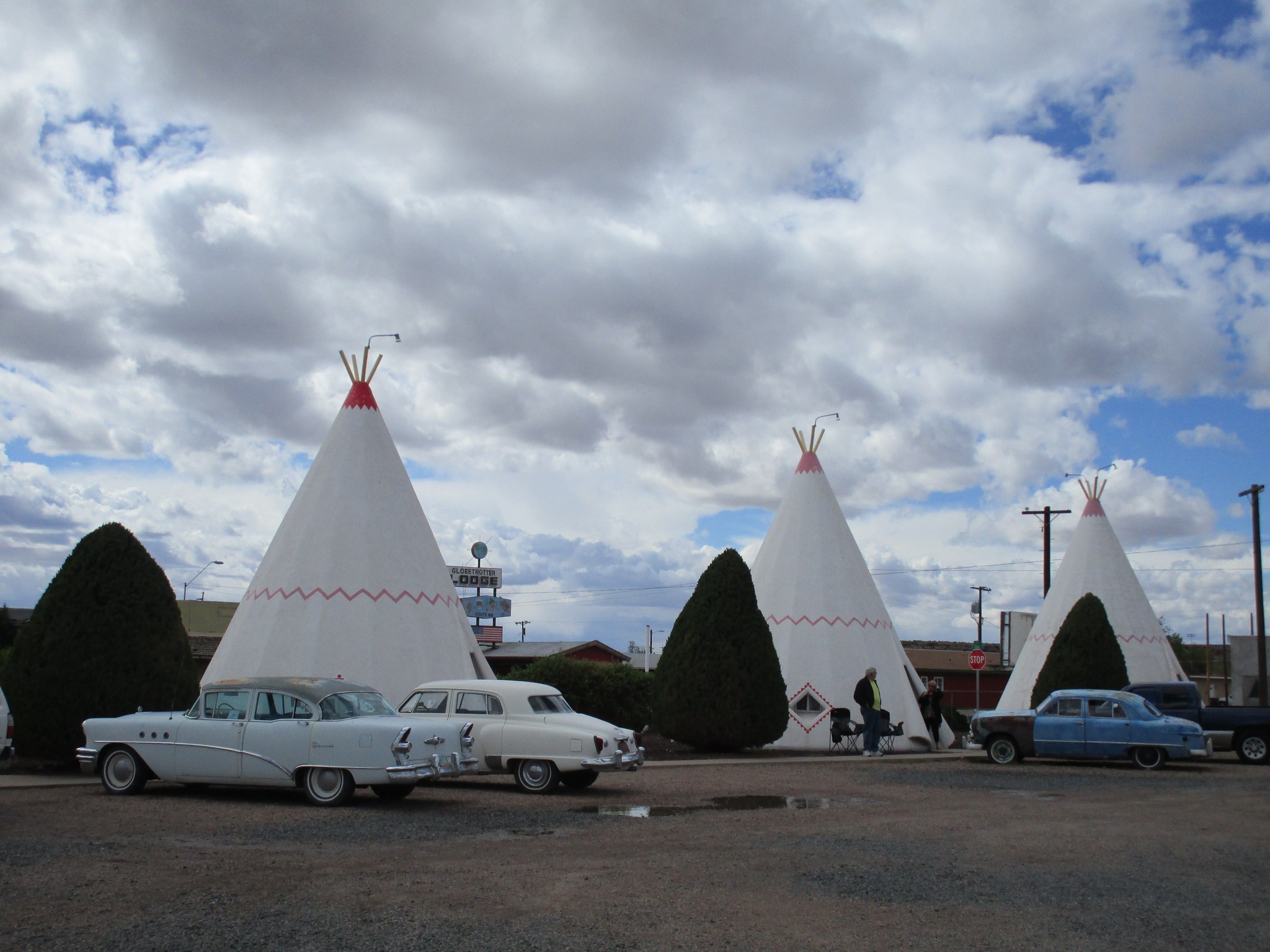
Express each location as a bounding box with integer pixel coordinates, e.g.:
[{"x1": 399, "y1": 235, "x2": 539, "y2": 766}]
[
  {"x1": 854, "y1": 667, "x2": 886, "y2": 757},
  {"x1": 917, "y1": 680, "x2": 945, "y2": 750}
]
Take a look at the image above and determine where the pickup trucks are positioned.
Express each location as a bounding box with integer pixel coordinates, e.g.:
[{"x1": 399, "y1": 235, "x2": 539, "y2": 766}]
[{"x1": 1059, "y1": 681, "x2": 1270, "y2": 765}]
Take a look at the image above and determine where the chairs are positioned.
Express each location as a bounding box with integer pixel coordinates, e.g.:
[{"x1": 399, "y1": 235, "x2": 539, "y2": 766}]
[
  {"x1": 828, "y1": 707, "x2": 863, "y2": 750},
  {"x1": 880, "y1": 709, "x2": 905, "y2": 754}
]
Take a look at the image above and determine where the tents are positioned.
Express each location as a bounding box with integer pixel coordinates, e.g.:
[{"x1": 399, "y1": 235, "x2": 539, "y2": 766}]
[
  {"x1": 194, "y1": 381, "x2": 503, "y2": 719},
  {"x1": 995, "y1": 495, "x2": 1210, "y2": 750},
  {"x1": 739, "y1": 457, "x2": 958, "y2": 757}
]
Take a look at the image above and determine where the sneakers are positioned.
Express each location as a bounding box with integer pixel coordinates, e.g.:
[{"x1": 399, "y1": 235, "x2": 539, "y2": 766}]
[
  {"x1": 871, "y1": 751, "x2": 883, "y2": 756},
  {"x1": 862, "y1": 750, "x2": 871, "y2": 757}
]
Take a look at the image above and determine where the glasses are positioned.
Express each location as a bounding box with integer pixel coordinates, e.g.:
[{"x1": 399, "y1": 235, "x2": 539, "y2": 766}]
[{"x1": 929, "y1": 684, "x2": 934, "y2": 688}]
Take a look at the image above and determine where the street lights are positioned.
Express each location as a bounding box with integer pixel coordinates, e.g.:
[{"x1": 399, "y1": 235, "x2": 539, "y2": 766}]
[
  {"x1": 183, "y1": 561, "x2": 221, "y2": 601},
  {"x1": 650, "y1": 630, "x2": 664, "y2": 654},
  {"x1": 1186, "y1": 633, "x2": 1198, "y2": 676}
]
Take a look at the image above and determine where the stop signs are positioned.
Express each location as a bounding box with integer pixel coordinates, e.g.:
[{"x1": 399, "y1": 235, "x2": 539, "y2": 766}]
[{"x1": 969, "y1": 649, "x2": 986, "y2": 670}]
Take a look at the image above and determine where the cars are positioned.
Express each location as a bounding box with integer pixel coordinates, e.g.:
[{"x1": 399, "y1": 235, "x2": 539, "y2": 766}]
[
  {"x1": 75, "y1": 679, "x2": 478, "y2": 806},
  {"x1": 967, "y1": 689, "x2": 1212, "y2": 770},
  {"x1": 394, "y1": 682, "x2": 643, "y2": 794}
]
